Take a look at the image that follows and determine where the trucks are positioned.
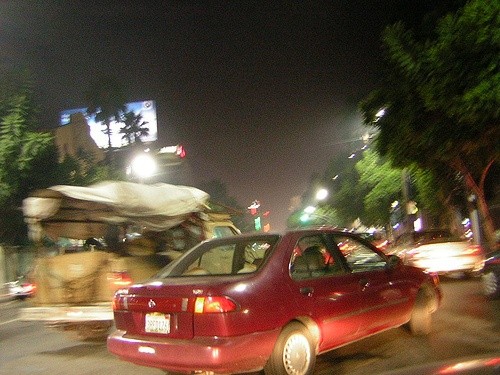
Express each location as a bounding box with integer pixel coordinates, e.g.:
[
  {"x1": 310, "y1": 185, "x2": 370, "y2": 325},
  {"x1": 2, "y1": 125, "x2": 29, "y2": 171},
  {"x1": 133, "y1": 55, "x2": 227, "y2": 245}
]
[{"x1": 21, "y1": 179, "x2": 261, "y2": 341}]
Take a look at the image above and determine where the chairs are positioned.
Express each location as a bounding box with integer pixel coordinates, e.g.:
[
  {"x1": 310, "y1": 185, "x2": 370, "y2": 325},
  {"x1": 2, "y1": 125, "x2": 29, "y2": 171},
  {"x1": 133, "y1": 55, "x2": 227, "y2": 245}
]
[
  {"x1": 237, "y1": 265, "x2": 256, "y2": 273},
  {"x1": 186, "y1": 268, "x2": 209, "y2": 275},
  {"x1": 328, "y1": 263, "x2": 356, "y2": 276},
  {"x1": 292, "y1": 254, "x2": 324, "y2": 278}
]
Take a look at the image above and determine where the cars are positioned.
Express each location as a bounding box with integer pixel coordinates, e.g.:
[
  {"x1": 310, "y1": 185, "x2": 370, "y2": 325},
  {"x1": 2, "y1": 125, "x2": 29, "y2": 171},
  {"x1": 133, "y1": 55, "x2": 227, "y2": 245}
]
[
  {"x1": 381, "y1": 230, "x2": 484, "y2": 280},
  {"x1": 12, "y1": 267, "x2": 38, "y2": 301},
  {"x1": 106, "y1": 227, "x2": 442, "y2": 375},
  {"x1": 480, "y1": 248, "x2": 500, "y2": 300}
]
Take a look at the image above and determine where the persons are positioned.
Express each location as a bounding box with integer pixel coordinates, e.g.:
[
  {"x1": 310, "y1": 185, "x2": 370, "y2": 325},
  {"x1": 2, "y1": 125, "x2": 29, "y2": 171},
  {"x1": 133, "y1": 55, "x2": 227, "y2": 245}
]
[{"x1": 304, "y1": 244, "x2": 331, "y2": 272}]
